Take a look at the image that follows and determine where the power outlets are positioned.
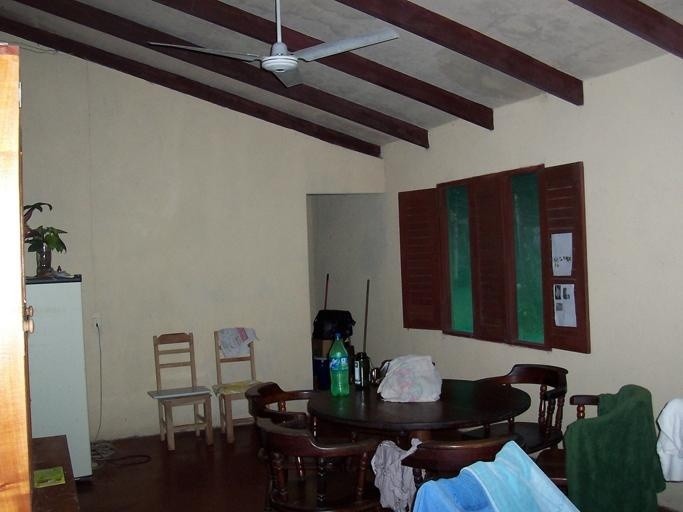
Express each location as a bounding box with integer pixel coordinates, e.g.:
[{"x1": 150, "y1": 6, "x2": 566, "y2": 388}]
[{"x1": 92, "y1": 318, "x2": 101, "y2": 330}]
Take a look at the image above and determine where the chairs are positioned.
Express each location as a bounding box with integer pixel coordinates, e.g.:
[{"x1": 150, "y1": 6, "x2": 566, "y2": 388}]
[{"x1": 152, "y1": 327, "x2": 263, "y2": 451}]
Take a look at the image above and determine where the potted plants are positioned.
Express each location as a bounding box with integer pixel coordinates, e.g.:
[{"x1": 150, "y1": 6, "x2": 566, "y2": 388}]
[{"x1": 22, "y1": 200, "x2": 67, "y2": 279}]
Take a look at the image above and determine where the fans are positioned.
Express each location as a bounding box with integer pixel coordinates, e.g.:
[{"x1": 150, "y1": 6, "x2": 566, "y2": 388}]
[{"x1": 147, "y1": 0, "x2": 400, "y2": 88}]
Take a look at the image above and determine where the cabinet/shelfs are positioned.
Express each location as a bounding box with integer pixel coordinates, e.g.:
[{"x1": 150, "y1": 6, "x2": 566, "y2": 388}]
[{"x1": 0, "y1": 45, "x2": 36, "y2": 512}]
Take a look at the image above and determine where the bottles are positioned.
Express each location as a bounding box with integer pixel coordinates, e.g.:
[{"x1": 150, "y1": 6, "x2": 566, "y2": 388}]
[
  {"x1": 354, "y1": 352, "x2": 370, "y2": 389},
  {"x1": 329, "y1": 332, "x2": 350, "y2": 397}
]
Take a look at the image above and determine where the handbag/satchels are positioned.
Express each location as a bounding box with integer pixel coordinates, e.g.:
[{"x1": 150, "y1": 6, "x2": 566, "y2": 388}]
[{"x1": 312, "y1": 309, "x2": 355, "y2": 340}]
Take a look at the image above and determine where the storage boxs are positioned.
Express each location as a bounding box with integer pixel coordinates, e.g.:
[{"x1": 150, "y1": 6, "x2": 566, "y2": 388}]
[{"x1": 311, "y1": 339, "x2": 354, "y2": 358}]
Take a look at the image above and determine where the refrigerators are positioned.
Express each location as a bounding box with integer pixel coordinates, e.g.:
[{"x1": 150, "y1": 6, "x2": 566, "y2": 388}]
[{"x1": 25, "y1": 273, "x2": 94, "y2": 481}]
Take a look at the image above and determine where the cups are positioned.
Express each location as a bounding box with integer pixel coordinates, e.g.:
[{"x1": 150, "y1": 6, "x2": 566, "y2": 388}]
[{"x1": 371, "y1": 367, "x2": 379, "y2": 381}]
